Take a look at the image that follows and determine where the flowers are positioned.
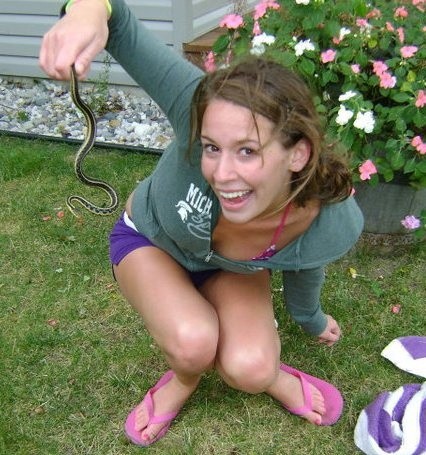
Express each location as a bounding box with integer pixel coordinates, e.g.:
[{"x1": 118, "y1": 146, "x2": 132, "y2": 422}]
[{"x1": 205, "y1": 0, "x2": 426, "y2": 228}]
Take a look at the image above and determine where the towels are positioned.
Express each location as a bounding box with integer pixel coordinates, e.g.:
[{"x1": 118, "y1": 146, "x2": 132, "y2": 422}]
[{"x1": 353, "y1": 335, "x2": 426, "y2": 455}]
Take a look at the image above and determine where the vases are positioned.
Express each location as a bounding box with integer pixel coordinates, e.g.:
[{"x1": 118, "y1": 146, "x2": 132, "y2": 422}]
[{"x1": 351, "y1": 182, "x2": 426, "y2": 235}]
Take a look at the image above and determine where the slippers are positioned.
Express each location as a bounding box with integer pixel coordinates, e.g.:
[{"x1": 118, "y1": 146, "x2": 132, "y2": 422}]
[
  {"x1": 280, "y1": 363, "x2": 343, "y2": 426},
  {"x1": 125, "y1": 370, "x2": 181, "y2": 446}
]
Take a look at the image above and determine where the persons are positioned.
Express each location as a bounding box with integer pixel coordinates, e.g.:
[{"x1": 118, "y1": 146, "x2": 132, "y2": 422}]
[{"x1": 38, "y1": 0, "x2": 364, "y2": 448}]
